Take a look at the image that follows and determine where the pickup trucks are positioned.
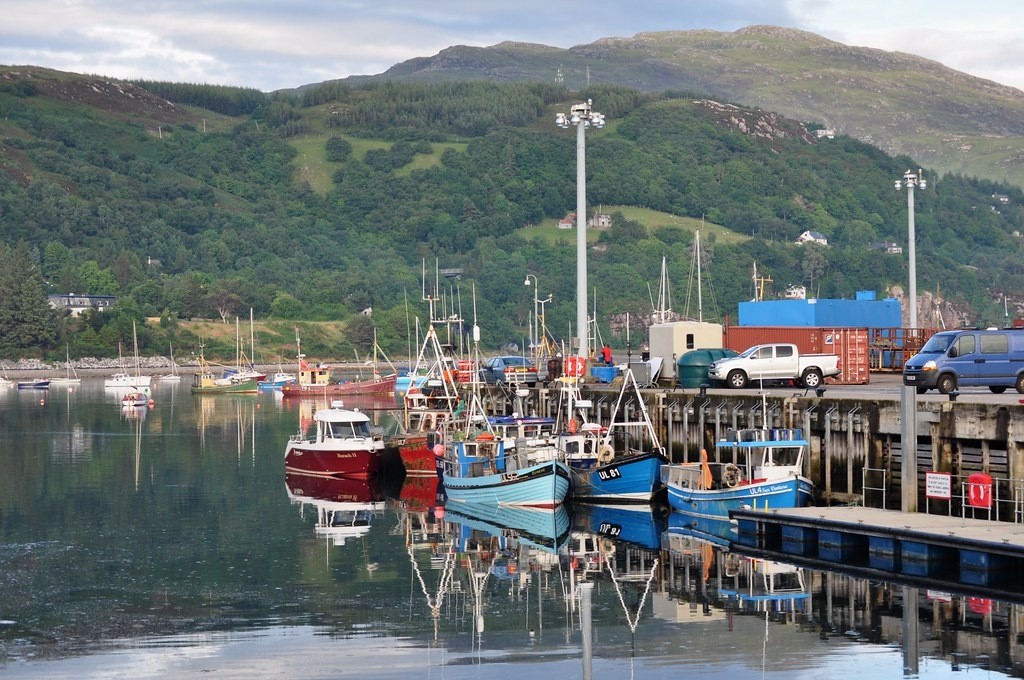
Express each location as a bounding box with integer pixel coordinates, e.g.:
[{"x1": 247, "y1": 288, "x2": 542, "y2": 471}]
[{"x1": 709, "y1": 342, "x2": 841, "y2": 389}]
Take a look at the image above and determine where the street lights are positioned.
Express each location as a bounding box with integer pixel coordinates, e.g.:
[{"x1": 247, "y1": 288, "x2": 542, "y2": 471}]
[
  {"x1": 894, "y1": 174, "x2": 927, "y2": 354},
  {"x1": 554, "y1": 100, "x2": 606, "y2": 365},
  {"x1": 525, "y1": 274, "x2": 540, "y2": 370}
]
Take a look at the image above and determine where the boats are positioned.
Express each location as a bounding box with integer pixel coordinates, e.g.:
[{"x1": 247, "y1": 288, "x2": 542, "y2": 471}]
[
  {"x1": 570, "y1": 494, "x2": 669, "y2": 658},
  {"x1": 16, "y1": 380, "x2": 51, "y2": 390},
  {"x1": 47, "y1": 342, "x2": 82, "y2": 384},
  {"x1": 571, "y1": 340, "x2": 671, "y2": 502},
  {"x1": 122, "y1": 392, "x2": 149, "y2": 406},
  {"x1": 0, "y1": 363, "x2": 13, "y2": 385},
  {"x1": 374, "y1": 257, "x2": 619, "y2": 468},
  {"x1": 433, "y1": 364, "x2": 570, "y2": 508},
  {"x1": 283, "y1": 471, "x2": 403, "y2": 569},
  {"x1": 187, "y1": 308, "x2": 396, "y2": 397},
  {"x1": 665, "y1": 511, "x2": 811, "y2": 680},
  {"x1": 443, "y1": 498, "x2": 572, "y2": 680},
  {"x1": 666, "y1": 346, "x2": 817, "y2": 520},
  {"x1": 281, "y1": 404, "x2": 385, "y2": 476}
]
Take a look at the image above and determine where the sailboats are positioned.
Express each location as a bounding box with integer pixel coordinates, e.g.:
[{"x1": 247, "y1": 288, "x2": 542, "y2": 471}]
[
  {"x1": 155, "y1": 341, "x2": 181, "y2": 381},
  {"x1": 103, "y1": 319, "x2": 152, "y2": 388}
]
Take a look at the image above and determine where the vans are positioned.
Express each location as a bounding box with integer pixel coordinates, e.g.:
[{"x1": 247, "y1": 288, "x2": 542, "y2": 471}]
[{"x1": 901, "y1": 328, "x2": 1024, "y2": 395}]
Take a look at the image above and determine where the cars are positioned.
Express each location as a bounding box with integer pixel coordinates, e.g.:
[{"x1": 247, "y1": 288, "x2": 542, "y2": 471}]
[{"x1": 479, "y1": 355, "x2": 538, "y2": 388}]
